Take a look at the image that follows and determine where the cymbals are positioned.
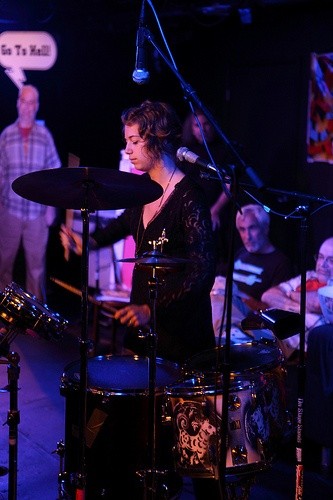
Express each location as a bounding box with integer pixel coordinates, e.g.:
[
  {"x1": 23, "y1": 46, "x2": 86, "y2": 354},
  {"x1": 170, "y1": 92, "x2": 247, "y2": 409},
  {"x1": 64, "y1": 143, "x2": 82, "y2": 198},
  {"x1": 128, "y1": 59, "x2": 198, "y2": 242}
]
[
  {"x1": 11, "y1": 166, "x2": 165, "y2": 213},
  {"x1": 115, "y1": 249, "x2": 190, "y2": 267}
]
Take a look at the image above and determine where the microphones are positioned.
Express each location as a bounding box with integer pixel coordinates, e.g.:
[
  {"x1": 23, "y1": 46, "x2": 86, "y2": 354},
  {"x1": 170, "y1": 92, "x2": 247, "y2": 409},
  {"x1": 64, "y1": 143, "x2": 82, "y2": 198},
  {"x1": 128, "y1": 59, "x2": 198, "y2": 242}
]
[
  {"x1": 131, "y1": 0, "x2": 149, "y2": 85},
  {"x1": 177, "y1": 147, "x2": 231, "y2": 180}
]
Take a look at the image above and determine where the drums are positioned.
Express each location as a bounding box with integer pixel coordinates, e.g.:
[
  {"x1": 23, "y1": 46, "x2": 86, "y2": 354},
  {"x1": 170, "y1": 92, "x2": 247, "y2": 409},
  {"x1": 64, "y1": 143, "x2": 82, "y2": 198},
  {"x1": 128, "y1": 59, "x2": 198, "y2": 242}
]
[
  {"x1": 183, "y1": 338, "x2": 292, "y2": 442},
  {"x1": 169, "y1": 375, "x2": 269, "y2": 480},
  {"x1": 1, "y1": 281, "x2": 70, "y2": 341},
  {"x1": 57, "y1": 353, "x2": 183, "y2": 500}
]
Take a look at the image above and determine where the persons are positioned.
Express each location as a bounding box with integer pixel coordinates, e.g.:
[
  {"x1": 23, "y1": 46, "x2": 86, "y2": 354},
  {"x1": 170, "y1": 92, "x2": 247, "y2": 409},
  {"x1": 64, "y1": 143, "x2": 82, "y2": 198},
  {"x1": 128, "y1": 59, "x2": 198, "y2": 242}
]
[{"x1": 2, "y1": 83, "x2": 332, "y2": 415}]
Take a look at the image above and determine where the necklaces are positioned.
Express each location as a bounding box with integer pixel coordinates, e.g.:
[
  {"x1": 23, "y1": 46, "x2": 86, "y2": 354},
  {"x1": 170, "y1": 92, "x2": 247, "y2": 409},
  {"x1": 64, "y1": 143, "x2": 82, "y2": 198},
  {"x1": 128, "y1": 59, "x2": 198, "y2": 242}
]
[{"x1": 137, "y1": 164, "x2": 177, "y2": 255}]
